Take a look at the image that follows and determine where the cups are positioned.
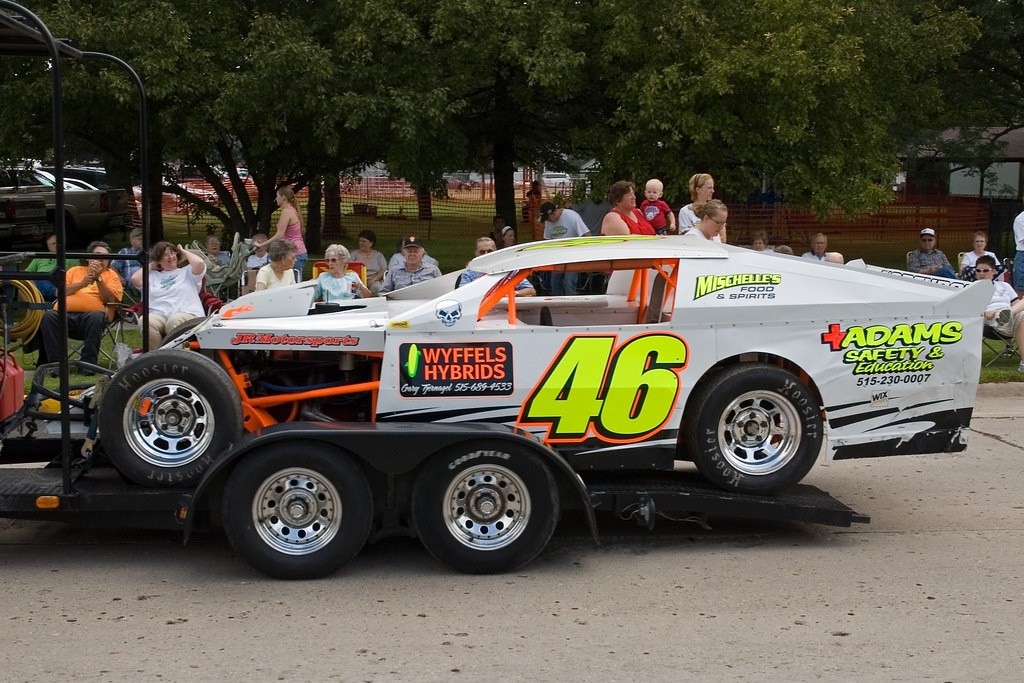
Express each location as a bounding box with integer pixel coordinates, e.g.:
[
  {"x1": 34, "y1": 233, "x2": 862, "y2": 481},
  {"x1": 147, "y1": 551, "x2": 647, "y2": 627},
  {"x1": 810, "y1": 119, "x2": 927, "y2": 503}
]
[{"x1": 244, "y1": 238, "x2": 253, "y2": 245}]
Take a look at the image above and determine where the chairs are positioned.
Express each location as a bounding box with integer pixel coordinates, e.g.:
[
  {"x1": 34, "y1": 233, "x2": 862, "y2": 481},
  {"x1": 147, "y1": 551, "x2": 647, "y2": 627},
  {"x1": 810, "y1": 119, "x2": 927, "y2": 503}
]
[
  {"x1": 313, "y1": 262, "x2": 367, "y2": 291},
  {"x1": 982, "y1": 324, "x2": 1021, "y2": 368},
  {"x1": 238, "y1": 270, "x2": 300, "y2": 292},
  {"x1": 52, "y1": 283, "x2": 142, "y2": 369},
  {"x1": 647, "y1": 271, "x2": 669, "y2": 325},
  {"x1": 122, "y1": 277, "x2": 222, "y2": 339}
]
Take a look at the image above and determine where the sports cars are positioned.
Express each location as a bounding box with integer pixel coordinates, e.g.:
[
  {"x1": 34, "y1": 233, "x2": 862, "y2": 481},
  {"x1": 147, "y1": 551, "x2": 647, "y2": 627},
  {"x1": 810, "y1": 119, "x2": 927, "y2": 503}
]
[{"x1": 95, "y1": 232, "x2": 999, "y2": 499}]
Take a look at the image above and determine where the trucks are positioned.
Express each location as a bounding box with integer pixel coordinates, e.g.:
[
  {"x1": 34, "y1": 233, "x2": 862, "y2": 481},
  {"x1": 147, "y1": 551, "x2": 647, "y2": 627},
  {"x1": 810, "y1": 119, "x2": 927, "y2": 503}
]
[{"x1": 0, "y1": 1, "x2": 875, "y2": 583}]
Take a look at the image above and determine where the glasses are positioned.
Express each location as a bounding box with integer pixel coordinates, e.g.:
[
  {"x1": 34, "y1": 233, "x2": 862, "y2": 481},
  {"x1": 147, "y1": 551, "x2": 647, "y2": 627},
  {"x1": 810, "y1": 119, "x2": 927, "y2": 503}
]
[
  {"x1": 325, "y1": 257, "x2": 343, "y2": 263},
  {"x1": 477, "y1": 249, "x2": 494, "y2": 255},
  {"x1": 208, "y1": 241, "x2": 222, "y2": 246},
  {"x1": 708, "y1": 214, "x2": 728, "y2": 227},
  {"x1": 921, "y1": 238, "x2": 935, "y2": 243},
  {"x1": 976, "y1": 268, "x2": 994, "y2": 272}
]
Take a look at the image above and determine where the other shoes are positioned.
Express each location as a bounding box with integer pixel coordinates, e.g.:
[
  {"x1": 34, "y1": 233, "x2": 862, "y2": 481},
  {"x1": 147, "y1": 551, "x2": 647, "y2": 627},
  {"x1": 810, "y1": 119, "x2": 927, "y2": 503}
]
[
  {"x1": 995, "y1": 310, "x2": 1012, "y2": 326},
  {"x1": 51, "y1": 369, "x2": 60, "y2": 377},
  {"x1": 78, "y1": 367, "x2": 95, "y2": 376},
  {"x1": 1017, "y1": 360, "x2": 1024, "y2": 374}
]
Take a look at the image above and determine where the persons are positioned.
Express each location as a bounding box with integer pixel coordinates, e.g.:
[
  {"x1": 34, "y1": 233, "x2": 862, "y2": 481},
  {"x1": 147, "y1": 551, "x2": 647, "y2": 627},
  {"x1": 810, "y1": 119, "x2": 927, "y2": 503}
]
[
  {"x1": 751, "y1": 227, "x2": 1008, "y2": 285},
  {"x1": 313, "y1": 229, "x2": 443, "y2": 302},
  {"x1": 538, "y1": 173, "x2": 728, "y2": 296},
  {"x1": 22, "y1": 227, "x2": 231, "y2": 378},
  {"x1": 1013, "y1": 210, "x2": 1024, "y2": 298},
  {"x1": 459, "y1": 213, "x2": 536, "y2": 297},
  {"x1": 522, "y1": 180, "x2": 542, "y2": 222},
  {"x1": 975, "y1": 255, "x2": 1024, "y2": 373},
  {"x1": 244, "y1": 186, "x2": 308, "y2": 291}
]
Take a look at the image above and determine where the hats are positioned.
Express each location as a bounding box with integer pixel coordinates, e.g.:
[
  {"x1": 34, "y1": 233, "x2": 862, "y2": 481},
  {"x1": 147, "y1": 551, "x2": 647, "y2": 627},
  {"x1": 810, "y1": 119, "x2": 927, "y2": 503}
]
[
  {"x1": 540, "y1": 202, "x2": 556, "y2": 223},
  {"x1": 404, "y1": 236, "x2": 421, "y2": 248},
  {"x1": 920, "y1": 227, "x2": 937, "y2": 237}
]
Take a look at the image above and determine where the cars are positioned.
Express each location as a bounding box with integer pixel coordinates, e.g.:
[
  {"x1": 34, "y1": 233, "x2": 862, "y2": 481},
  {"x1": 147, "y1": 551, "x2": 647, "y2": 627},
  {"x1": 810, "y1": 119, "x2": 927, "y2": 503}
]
[
  {"x1": 470, "y1": 174, "x2": 520, "y2": 191},
  {"x1": 0, "y1": 158, "x2": 256, "y2": 253},
  {"x1": 442, "y1": 176, "x2": 471, "y2": 192},
  {"x1": 520, "y1": 171, "x2": 572, "y2": 189}
]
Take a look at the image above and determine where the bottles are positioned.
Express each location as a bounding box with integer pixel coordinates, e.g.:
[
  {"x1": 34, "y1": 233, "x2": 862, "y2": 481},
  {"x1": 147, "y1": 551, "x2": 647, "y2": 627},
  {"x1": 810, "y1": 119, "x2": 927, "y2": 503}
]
[{"x1": 351, "y1": 282, "x2": 358, "y2": 299}]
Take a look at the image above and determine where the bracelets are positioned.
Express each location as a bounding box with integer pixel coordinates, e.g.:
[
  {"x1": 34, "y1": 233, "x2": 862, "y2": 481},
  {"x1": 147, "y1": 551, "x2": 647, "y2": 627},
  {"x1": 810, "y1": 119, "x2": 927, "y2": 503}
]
[{"x1": 359, "y1": 282, "x2": 362, "y2": 291}]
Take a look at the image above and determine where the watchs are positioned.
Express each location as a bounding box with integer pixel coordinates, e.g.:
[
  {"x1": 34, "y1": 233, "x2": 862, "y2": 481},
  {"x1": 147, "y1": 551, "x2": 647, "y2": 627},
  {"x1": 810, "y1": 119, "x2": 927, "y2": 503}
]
[{"x1": 95, "y1": 277, "x2": 104, "y2": 282}]
